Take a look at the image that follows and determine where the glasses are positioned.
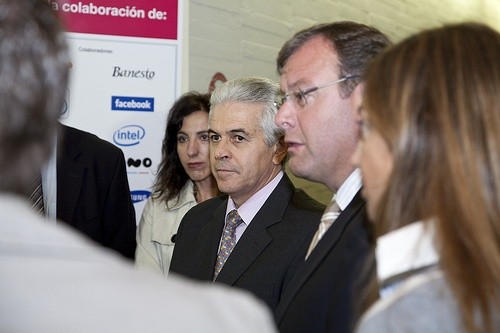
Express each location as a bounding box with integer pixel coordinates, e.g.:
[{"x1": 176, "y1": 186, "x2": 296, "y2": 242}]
[{"x1": 275, "y1": 73, "x2": 360, "y2": 108}]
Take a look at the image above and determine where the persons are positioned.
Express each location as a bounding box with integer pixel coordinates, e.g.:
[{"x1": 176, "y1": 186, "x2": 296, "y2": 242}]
[
  {"x1": 23, "y1": 120, "x2": 137, "y2": 262},
  {"x1": 274, "y1": 21, "x2": 396, "y2": 333},
  {"x1": 167, "y1": 76, "x2": 327, "y2": 311},
  {"x1": 0, "y1": 0, "x2": 278, "y2": 332},
  {"x1": 134, "y1": 89, "x2": 225, "y2": 278},
  {"x1": 354, "y1": 20, "x2": 500, "y2": 333}
]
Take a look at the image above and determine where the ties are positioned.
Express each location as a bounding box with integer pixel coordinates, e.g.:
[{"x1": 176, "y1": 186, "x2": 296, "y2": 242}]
[
  {"x1": 305, "y1": 200, "x2": 341, "y2": 259},
  {"x1": 25, "y1": 167, "x2": 47, "y2": 220},
  {"x1": 212, "y1": 210, "x2": 243, "y2": 282}
]
